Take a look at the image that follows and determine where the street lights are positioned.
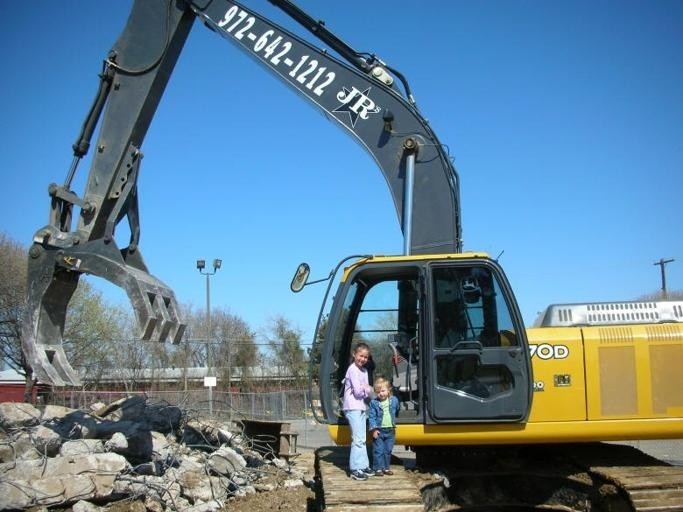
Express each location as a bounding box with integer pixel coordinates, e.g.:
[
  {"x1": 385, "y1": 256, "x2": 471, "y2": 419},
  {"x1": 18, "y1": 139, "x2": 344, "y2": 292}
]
[{"x1": 196, "y1": 258, "x2": 223, "y2": 377}]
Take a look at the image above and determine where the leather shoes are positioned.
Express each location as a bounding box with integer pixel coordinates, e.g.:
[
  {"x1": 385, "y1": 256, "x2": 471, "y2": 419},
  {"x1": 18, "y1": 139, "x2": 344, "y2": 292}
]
[
  {"x1": 375, "y1": 469, "x2": 383, "y2": 476},
  {"x1": 382, "y1": 469, "x2": 392, "y2": 475}
]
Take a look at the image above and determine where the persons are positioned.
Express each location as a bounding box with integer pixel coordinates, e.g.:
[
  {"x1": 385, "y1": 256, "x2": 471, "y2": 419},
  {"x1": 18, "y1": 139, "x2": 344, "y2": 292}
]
[
  {"x1": 342, "y1": 342, "x2": 376, "y2": 481},
  {"x1": 368, "y1": 378, "x2": 400, "y2": 476}
]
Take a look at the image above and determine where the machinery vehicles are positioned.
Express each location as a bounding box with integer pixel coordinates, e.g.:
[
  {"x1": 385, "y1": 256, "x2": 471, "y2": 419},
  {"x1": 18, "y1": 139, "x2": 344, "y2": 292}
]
[{"x1": 13, "y1": 1, "x2": 681, "y2": 482}]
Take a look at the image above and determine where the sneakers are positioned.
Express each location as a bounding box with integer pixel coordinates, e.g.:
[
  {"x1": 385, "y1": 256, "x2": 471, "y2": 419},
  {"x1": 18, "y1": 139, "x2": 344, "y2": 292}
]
[
  {"x1": 349, "y1": 469, "x2": 367, "y2": 481},
  {"x1": 363, "y1": 467, "x2": 376, "y2": 476}
]
